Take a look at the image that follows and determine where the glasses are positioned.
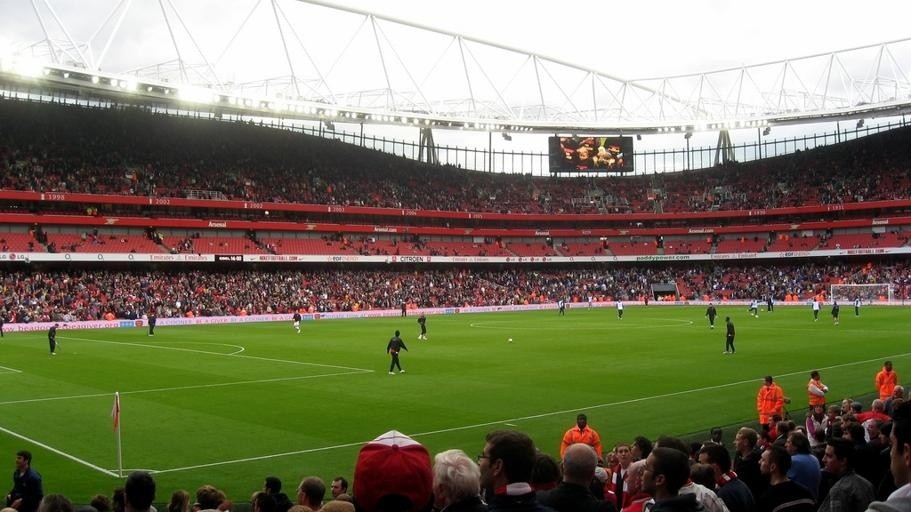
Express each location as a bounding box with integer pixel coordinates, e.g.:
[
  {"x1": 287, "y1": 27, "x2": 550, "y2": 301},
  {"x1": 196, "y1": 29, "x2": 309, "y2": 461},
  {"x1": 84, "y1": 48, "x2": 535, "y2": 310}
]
[{"x1": 476, "y1": 455, "x2": 491, "y2": 461}]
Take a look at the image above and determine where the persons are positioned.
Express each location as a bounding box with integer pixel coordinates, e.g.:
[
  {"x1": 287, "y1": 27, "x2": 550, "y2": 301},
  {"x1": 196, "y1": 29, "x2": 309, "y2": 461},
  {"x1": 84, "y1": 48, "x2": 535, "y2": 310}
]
[
  {"x1": 1, "y1": 97, "x2": 911, "y2": 338},
  {"x1": 722, "y1": 316, "x2": 736, "y2": 354},
  {"x1": 386, "y1": 330, "x2": 407, "y2": 374},
  {"x1": 48, "y1": 324, "x2": 58, "y2": 355},
  {"x1": 0, "y1": 361, "x2": 911, "y2": 512}
]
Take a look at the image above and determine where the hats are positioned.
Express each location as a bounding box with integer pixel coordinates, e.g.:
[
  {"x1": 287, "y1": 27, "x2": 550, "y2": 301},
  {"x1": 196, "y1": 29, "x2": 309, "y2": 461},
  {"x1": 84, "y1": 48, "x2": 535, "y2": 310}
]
[{"x1": 353, "y1": 430, "x2": 435, "y2": 510}]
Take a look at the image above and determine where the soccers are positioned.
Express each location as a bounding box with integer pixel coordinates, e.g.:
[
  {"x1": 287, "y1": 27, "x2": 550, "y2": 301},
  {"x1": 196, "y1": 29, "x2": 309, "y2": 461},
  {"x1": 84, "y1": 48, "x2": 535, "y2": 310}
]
[{"x1": 507, "y1": 338, "x2": 512, "y2": 343}]
